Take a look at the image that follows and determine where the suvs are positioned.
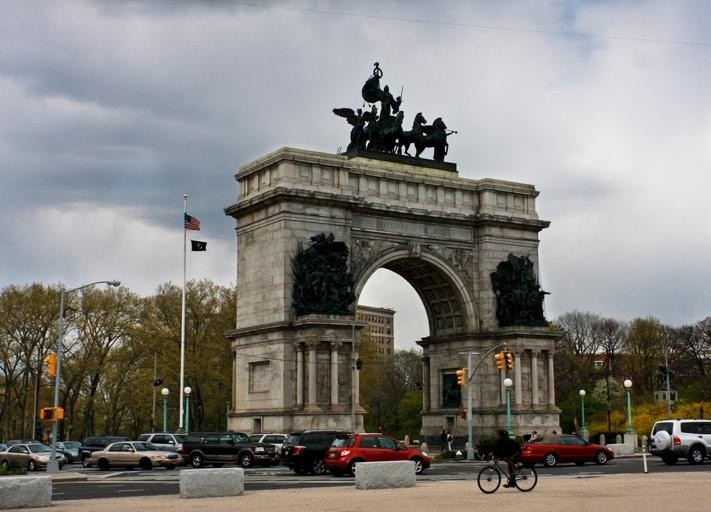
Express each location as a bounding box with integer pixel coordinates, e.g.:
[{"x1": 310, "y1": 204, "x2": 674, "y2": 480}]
[{"x1": 649, "y1": 416, "x2": 711, "y2": 466}]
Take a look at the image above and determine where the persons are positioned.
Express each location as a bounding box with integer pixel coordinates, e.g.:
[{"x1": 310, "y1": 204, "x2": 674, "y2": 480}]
[
  {"x1": 447, "y1": 431, "x2": 453, "y2": 451},
  {"x1": 495, "y1": 430, "x2": 522, "y2": 487},
  {"x1": 440, "y1": 430, "x2": 448, "y2": 452},
  {"x1": 529, "y1": 431, "x2": 537, "y2": 441}
]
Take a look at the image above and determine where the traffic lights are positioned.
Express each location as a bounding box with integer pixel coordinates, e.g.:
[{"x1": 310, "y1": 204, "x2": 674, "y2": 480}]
[
  {"x1": 495, "y1": 353, "x2": 505, "y2": 371},
  {"x1": 457, "y1": 369, "x2": 465, "y2": 385},
  {"x1": 56, "y1": 408, "x2": 63, "y2": 420},
  {"x1": 42, "y1": 408, "x2": 56, "y2": 421},
  {"x1": 506, "y1": 352, "x2": 512, "y2": 370}
]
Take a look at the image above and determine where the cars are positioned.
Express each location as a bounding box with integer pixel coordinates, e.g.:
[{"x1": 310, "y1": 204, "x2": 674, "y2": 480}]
[{"x1": 523, "y1": 433, "x2": 616, "y2": 465}]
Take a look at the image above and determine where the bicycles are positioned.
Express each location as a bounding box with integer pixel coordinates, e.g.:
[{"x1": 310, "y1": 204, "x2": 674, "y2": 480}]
[{"x1": 476, "y1": 454, "x2": 539, "y2": 495}]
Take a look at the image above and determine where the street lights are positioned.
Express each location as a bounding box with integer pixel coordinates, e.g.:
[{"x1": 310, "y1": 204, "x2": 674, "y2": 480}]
[
  {"x1": 159, "y1": 388, "x2": 171, "y2": 435},
  {"x1": 665, "y1": 345, "x2": 674, "y2": 407},
  {"x1": 50, "y1": 276, "x2": 122, "y2": 474},
  {"x1": 579, "y1": 388, "x2": 590, "y2": 431},
  {"x1": 623, "y1": 379, "x2": 635, "y2": 433},
  {"x1": 503, "y1": 377, "x2": 516, "y2": 439},
  {"x1": 183, "y1": 386, "x2": 193, "y2": 430}
]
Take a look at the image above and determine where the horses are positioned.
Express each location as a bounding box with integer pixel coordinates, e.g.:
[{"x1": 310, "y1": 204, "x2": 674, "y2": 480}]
[{"x1": 396, "y1": 113, "x2": 449, "y2": 157}]
